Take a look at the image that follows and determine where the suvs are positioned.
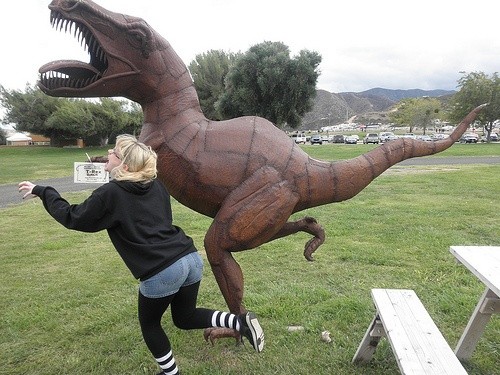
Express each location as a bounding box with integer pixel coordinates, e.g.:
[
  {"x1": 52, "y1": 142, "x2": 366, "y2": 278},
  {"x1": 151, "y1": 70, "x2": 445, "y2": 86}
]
[
  {"x1": 379, "y1": 132, "x2": 398, "y2": 143},
  {"x1": 363, "y1": 133, "x2": 380, "y2": 145},
  {"x1": 333, "y1": 135, "x2": 345, "y2": 143}
]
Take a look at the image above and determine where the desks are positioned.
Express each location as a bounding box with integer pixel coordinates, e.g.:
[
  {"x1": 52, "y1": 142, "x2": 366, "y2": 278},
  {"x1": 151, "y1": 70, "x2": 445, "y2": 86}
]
[{"x1": 449, "y1": 245, "x2": 500, "y2": 360}]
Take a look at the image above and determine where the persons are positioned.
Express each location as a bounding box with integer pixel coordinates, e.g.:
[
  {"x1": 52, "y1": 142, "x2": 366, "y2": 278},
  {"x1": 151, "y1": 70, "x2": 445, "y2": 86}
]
[{"x1": 19, "y1": 133, "x2": 265, "y2": 375}]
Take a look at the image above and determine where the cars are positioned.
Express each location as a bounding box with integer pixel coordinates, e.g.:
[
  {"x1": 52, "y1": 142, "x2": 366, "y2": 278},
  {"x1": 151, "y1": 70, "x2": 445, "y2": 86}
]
[
  {"x1": 402, "y1": 133, "x2": 479, "y2": 144},
  {"x1": 345, "y1": 136, "x2": 357, "y2": 144},
  {"x1": 321, "y1": 135, "x2": 334, "y2": 142},
  {"x1": 351, "y1": 134, "x2": 360, "y2": 141},
  {"x1": 481, "y1": 132, "x2": 498, "y2": 143},
  {"x1": 306, "y1": 136, "x2": 311, "y2": 142},
  {"x1": 310, "y1": 135, "x2": 323, "y2": 145}
]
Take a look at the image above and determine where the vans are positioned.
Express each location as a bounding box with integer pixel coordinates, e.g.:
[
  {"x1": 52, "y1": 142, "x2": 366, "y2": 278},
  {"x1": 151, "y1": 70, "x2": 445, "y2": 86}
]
[{"x1": 288, "y1": 133, "x2": 306, "y2": 145}]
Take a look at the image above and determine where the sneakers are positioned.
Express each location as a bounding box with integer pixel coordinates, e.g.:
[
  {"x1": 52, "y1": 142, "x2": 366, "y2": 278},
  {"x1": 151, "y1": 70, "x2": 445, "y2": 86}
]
[
  {"x1": 156, "y1": 370, "x2": 181, "y2": 375},
  {"x1": 236, "y1": 309, "x2": 265, "y2": 354}
]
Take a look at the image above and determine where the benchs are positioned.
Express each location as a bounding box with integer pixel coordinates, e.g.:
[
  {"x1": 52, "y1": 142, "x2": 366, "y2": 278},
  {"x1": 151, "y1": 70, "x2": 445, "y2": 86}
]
[{"x1": 352, "y1": 288, "x2": 468, "y2": 375}]
